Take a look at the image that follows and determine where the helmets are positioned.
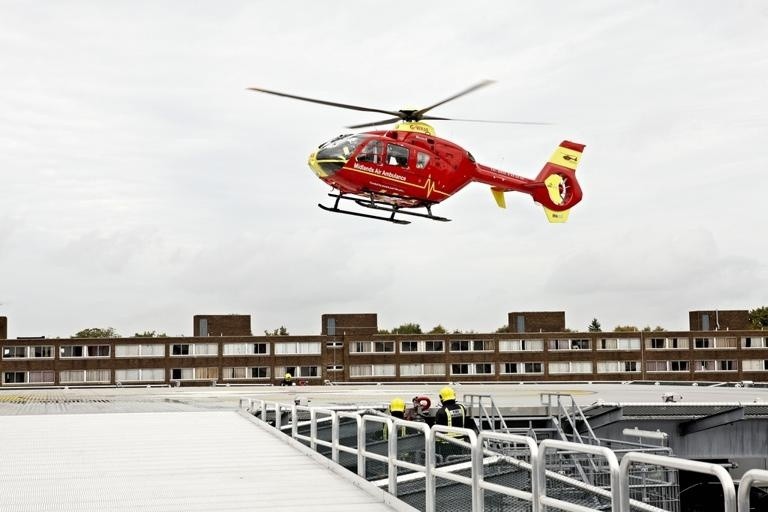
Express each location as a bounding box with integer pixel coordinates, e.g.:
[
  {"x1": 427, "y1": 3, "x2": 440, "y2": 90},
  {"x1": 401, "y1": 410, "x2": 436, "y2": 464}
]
[
  {"x1": 285, "y1": 373, "x2": 291, "y2": 381},
  {"x1": 389, "y1": 398, "x2": 406, "y2": 413},
  {"x1": 439, "y1": 388, "x2": 456, "y2": 402}
]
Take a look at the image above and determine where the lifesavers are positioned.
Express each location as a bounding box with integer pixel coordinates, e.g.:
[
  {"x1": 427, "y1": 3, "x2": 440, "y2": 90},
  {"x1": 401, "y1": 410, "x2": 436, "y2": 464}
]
[{"x1": 416, "y1": 396, "x2": 431, "y2": 410}]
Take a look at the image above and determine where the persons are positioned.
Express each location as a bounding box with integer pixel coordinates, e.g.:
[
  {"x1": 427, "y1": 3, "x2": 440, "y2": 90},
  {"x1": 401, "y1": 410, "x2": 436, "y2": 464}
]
[
  {"x1": 377, "y1": 399, "x2": 418, "y2": 475},
  {"x1": 357, "y1": 141, "x2": 380, "y2": 163},
  {"x1": 280, "y1": 373, "x2": 293, "y2": 386},
  {"x1": 436, "y1": 387, "x2": 467, "y2": 463},
  {"x1": 406, "y1": 397, "x2": 429, "y2": 422}
]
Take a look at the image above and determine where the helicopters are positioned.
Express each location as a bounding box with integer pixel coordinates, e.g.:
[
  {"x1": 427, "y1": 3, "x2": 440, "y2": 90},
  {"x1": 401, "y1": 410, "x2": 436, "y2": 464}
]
[{"x1": 246, "y1": 78, "x2": 585, "y2": 225}]
